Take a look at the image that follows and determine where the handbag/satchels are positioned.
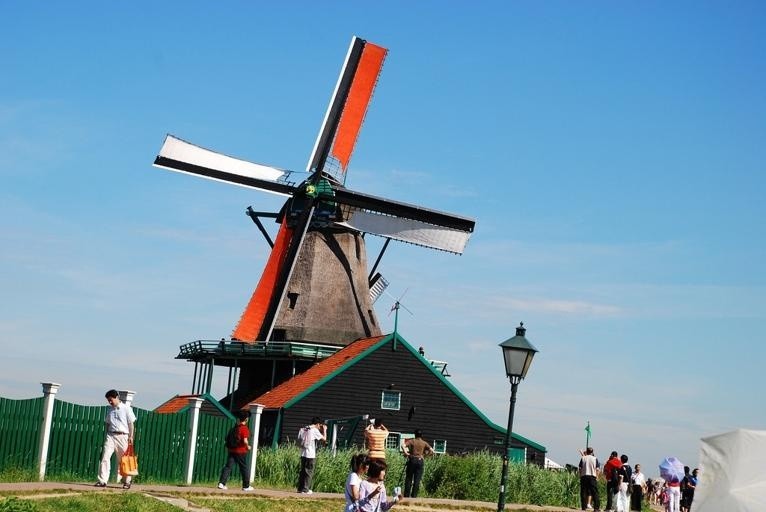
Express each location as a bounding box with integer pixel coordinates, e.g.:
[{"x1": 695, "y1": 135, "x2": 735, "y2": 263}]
[
  {"x1": 120, "y1": 444, "x2": 139, "y2": 476},
  {"x1": 628, "y1": 483, "x2": 634, "y2": 494}
]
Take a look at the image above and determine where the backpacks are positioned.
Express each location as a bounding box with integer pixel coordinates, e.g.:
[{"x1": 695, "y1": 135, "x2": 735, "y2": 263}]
[
  {"x1": 296, "y1": 425, "x2": 313, "y2": 448},
  {"x1": 226, "y1": 424, "x2": 245, "y2": 449}
]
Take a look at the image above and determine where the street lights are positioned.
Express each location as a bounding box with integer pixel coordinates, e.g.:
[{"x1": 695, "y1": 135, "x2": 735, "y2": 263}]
[{"x1": 490, "y1": 321, "x2": 538, "y2": 512}]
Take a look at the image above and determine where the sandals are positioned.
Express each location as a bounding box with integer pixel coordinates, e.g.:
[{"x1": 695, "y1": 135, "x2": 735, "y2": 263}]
[
  {"x1": 94, "y1": 482, "x2": 106, "y2": 487},
  {"x1": 123, "y1": 482, "x2": 132, "y2": 490}
]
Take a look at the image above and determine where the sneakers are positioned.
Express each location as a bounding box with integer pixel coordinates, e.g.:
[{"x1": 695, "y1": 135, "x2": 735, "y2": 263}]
[
  {"x1": 298, "y1": 489, "x2": 313, "y2": 494},
  {"x1": 218, "y1": 483, "x2": 228, "y2": 490},
  {"x1": 242, "y1": 486, "x2": 255, "y2": 491}
]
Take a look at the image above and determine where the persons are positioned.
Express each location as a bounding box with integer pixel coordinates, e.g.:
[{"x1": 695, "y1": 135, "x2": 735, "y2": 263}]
[
  {"x1": 359, "y1": 459, "x2": 403, "y2": 512},
  {"x1": 400, "y1": 428, "x2": 435, "y2": 497},
  {"x1": 577, "y1": 444, "x2": 701, "y2": 512},
  {"x1": 343, "y1": 453, "x2": 371, "y2": 512},
  {"x1": 217, "y1": 410, "x2": 255, "y2": 491},
  {"x1": 362, "y1": 416, "x2": 390, "y2": 468},
  {"x1": 296, "y1": 416, "x2": 328, "y2": 494},
  {"x1": 94, "y1": 389, "x2": 136, "y2": 489}
]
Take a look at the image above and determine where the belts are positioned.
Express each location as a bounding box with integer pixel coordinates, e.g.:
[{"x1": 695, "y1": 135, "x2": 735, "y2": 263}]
[{"x1": 108, "y1": 431, "x2": 128, "y2": 435}]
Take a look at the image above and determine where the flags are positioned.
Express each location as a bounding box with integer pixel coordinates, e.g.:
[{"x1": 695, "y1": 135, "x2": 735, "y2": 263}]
[{"x1": 585, "y1": 425, "x2": 593, "y2": 438}]
